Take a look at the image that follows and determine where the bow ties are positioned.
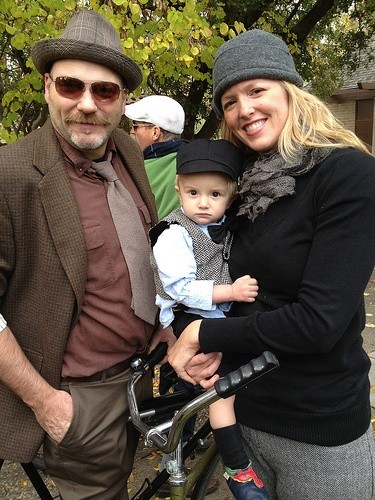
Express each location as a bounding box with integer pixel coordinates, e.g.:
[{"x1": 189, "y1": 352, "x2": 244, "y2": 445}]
[{"x1": 207, "y1": 215, "x2": 240, "y2": 244}]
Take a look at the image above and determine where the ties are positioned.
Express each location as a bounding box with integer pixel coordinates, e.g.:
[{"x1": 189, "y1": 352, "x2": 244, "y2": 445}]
[{"x1": 90, "y1": 154, "x2": 159, "y2": 327}]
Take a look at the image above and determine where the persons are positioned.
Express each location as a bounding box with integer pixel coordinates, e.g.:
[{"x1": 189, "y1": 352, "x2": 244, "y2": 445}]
[
  {"x1": 0, "y1": 10, "x2": 158, "y2": 500},
  {"x1": 212, "y1": 29, "x2": 375, "y2": 500},
  {"x1": 147, "y1": 138, "x2": 268, "y2": 500},
  {"x1": 124, "y1": 95, "x2": 185, "y2": 223}
]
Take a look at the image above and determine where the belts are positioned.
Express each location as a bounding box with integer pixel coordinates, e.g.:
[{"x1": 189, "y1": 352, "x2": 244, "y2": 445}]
[{"x1": 61, "y1": 355, "x2": 137, "y2": 382}]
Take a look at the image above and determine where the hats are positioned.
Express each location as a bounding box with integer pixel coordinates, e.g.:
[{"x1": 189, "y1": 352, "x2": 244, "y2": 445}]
[
  {"x1": 212, "y1": 29, "x2": 303, "y2": 119},
  {"x1": 124, "y1": 95, "x2": 185, "y2": 135},
  {"x1": 176, "y1": 139, "x2": 247, "y2": 181},
  {"x1": 31, "y1": 9, "x2": 143, "y2": 94}
]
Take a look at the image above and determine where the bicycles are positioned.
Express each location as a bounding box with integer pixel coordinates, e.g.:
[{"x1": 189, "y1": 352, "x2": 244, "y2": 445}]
[{"x1": 125, "y1": 340, "x2": 282, "y2": 499}]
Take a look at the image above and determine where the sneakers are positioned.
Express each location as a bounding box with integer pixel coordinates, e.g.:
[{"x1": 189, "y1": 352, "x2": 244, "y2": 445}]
[{"x1": 225, "y1": 460, "x2": 271, "y2": 500}]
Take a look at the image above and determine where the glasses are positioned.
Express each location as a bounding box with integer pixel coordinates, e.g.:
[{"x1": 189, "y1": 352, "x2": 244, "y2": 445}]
[
  {"x1": 130, "y1": 120, "x2": 154, "y2": 131},
  {"x1": 49, "y1": 74, "x2": 126, "y2": 103}
]
